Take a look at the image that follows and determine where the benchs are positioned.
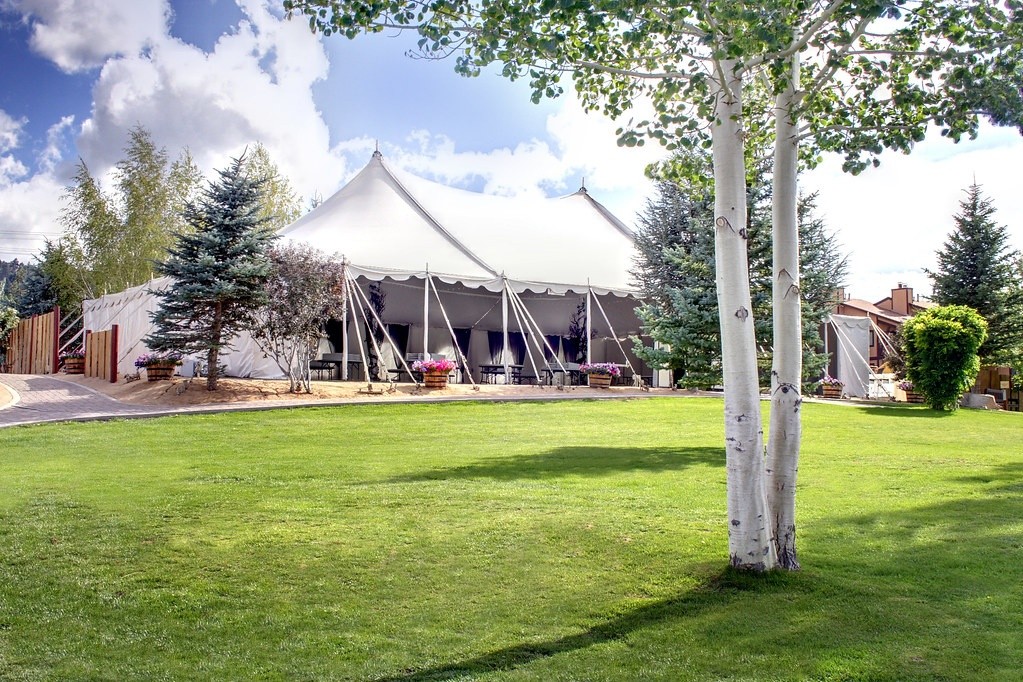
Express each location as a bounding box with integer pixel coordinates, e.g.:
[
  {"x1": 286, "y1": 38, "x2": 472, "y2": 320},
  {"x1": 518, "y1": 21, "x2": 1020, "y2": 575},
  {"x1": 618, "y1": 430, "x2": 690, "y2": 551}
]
[
  {"x1": 511, "y1": 375, "x2": 544, "y2": 384},
  {"x1": 480, "y1": 371, "x2": 521, "y2": 384},
  {"x1": 306, "y1": 366, "x2": 334, "y2": 380},
  {"x1": 387, "y1": 369, "x2": 456, "y2": 384}
]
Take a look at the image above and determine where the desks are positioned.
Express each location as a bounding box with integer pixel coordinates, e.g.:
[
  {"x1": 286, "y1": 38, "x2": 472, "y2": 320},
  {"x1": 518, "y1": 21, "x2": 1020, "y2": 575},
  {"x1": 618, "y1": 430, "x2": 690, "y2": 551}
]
[
  {"x1": 405, "y1": 360, "x2": 458, "y2": 383},
  {"x1": 541, "y1": 369, "x2": 584, "y2": 386},
  {"x1": 316, "y1": 360, "x2": 363, "y2": 380},
  {"x1": 618, "y1": 376, "x2": 653, "y2": 388},
  {"x1": 478, "y1": 364, "x2": 524, "y2": 384}
]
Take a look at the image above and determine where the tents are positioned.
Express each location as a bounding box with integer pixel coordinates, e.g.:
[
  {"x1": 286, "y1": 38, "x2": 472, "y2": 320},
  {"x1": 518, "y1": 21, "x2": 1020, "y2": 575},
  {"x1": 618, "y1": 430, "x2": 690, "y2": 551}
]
[{"x1": 82, "y1": 137, "x2": 916, "y2": 403}]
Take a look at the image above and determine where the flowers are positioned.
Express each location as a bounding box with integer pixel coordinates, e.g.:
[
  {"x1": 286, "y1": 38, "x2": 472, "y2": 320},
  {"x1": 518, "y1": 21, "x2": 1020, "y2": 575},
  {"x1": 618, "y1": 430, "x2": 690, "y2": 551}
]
[
  {"x1": 135, "y1": 354, "x2": 184, "y2": 367},
  {"x1": 578, "y1": 361, "x2": 621, "y2": 379},
  {"x1": 819, "y1": 375, "x2": 846, "y2": 388},
  {"x1": 57, "y1": 350, "x2": 86, "y2": 361},
  {"x1": 897, "y1": 381, "x2": 915, "y2": 391}
]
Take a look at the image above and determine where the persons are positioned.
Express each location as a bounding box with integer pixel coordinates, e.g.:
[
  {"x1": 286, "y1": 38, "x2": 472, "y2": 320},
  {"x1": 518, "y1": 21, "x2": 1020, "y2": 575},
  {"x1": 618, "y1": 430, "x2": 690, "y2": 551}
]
[{"x1": 369, "y1": 335, "x2": 380, "y2": 382}]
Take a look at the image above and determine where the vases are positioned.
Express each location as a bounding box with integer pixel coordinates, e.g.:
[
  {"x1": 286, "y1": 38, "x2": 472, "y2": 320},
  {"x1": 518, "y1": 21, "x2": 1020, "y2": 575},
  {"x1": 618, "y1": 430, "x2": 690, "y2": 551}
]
[
  {"x1": 145, "y1": 361, "x2": 176, "y2": 381},
  {"x1": 589, "y1": 372, "x2": 612, "y2": 389},
  {"x1": 65, "y1": 358, "x2": 84, "y2": 374},
  {"x1": 822, "y1": 386, "x2": 842, "y2": 399},
  {"x1": 905, "y1": 390, "x2": 924, "y2": 403}
]
